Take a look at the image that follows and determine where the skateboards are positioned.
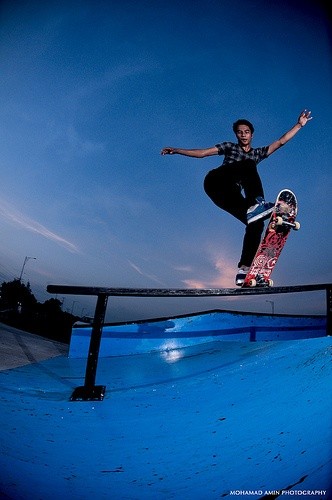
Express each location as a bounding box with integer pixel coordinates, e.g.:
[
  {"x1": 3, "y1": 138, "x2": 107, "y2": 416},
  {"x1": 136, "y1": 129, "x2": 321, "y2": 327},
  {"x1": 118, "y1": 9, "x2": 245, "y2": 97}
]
[{"x1": 242, "y1": 189, "x2": 300, "y2": 288}]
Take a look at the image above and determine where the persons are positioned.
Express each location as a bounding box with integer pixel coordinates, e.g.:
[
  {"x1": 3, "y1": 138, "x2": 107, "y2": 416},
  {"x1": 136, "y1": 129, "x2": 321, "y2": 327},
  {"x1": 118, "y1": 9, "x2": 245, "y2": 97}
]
[{"x1": 159, "y1": 109, "x2": 315, "y2": 286}]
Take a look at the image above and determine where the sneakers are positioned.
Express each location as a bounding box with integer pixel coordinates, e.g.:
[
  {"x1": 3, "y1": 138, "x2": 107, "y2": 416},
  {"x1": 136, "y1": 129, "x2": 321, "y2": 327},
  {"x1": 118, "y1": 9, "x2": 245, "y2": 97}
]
[
  {"x1": 235, "y1": 271, "x2": 246, "y2": 285},
  {"x1": 247, "y1": 196, "x2": 275, "y2": 225}
]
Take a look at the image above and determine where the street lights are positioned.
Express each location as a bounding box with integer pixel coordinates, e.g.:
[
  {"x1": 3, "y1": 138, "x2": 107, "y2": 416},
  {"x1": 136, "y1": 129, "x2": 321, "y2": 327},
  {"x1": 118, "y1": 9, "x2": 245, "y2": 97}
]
[{"x1": 19, "y1": 256, "x2": 37, "y2": 281}]
